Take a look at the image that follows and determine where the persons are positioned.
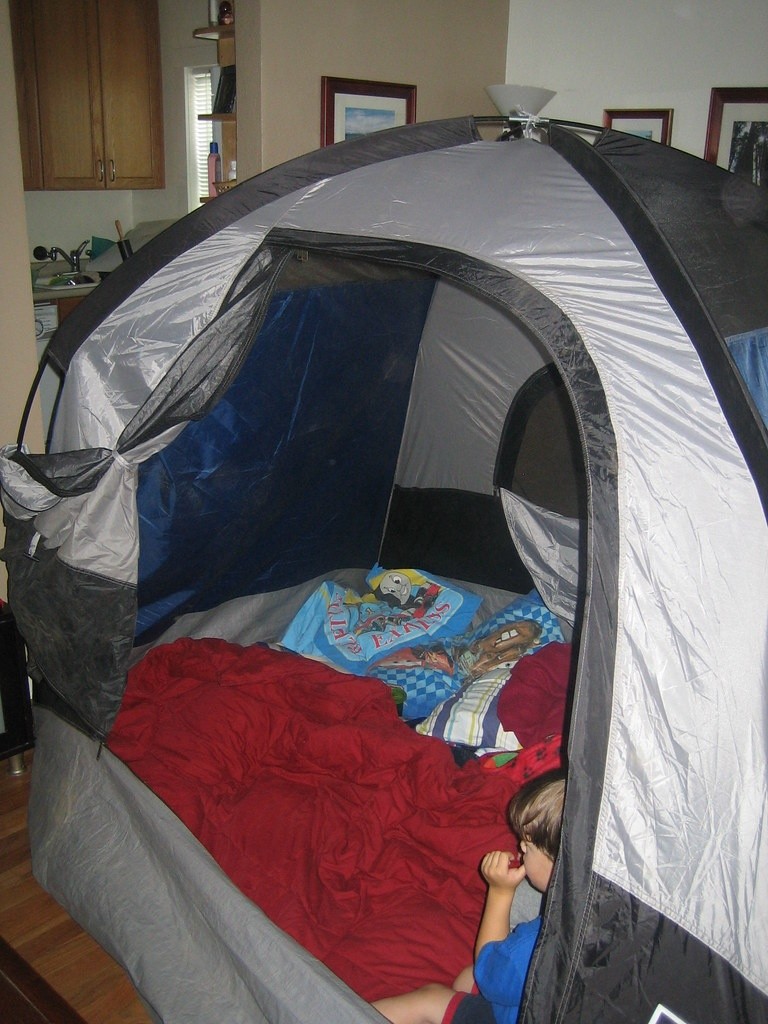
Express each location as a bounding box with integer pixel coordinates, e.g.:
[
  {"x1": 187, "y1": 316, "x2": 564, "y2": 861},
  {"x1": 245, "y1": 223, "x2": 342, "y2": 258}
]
[{"x1": 370, "y1": 768, "x2": 567, "y2": 1024}]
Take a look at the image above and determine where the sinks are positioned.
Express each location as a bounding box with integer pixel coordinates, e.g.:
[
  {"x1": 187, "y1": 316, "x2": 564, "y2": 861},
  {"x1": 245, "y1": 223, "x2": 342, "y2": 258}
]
[{"x1": 33, "y1": 271, "x2": 101, "y2": 291}]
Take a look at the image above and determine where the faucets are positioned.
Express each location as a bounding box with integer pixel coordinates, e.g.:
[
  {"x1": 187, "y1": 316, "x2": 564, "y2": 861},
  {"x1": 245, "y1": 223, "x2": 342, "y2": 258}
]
[{"x1": 46, "y1": 240, "x2": 91, "y2": 273}]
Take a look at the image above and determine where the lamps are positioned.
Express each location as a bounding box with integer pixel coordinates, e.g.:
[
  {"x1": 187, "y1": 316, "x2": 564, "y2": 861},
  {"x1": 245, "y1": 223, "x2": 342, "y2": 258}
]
[{"x1": 484, "y1": 82, "x2": 557, "y2": 115}]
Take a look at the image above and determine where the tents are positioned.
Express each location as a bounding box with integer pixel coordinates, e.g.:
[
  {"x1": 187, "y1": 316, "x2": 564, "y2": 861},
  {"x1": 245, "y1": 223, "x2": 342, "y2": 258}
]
[{"x1": 0, "y1": 112, "x2": 768, "y2": 1024}]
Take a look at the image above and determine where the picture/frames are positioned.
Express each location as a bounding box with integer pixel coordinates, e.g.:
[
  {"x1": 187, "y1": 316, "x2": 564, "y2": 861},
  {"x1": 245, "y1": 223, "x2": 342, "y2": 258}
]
[
  {"x1": 320, "y1": 75, "x2": 418, "y2": 146},
  {"x1": 602, "y1": 107, "x2": 674, "y2": 147},
  {"x1": 704, "y1": 85, "x2": 768, "y2": 191}
]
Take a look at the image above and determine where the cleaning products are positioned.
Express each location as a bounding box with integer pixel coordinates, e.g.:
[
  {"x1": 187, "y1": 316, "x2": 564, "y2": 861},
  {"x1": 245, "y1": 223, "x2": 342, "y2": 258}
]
[
  {"x1": 218, "y1": 2, "x2": 233, "y2": 25},
  {"x1": 207, "y1": 142, "x2": 221, "y2": 197},
  {"x1": 209, "y1": 0, "x2": 220, "y2": 26},
  {"x1": 228, "y1": 161, "x2": 238, "y2": 183}
]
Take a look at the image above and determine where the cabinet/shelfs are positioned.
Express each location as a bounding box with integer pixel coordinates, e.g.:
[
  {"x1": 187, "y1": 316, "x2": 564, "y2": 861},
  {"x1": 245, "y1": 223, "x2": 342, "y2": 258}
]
[
  {"x1": 31, "y1": 295, "x2": 87, "y2": 337},
  {"x1": 9, "y1": 0, "x2": 166, "y2": 192},
  {"x1": 192, "y1": 22, "x2": 236, "y2": 205}
]
